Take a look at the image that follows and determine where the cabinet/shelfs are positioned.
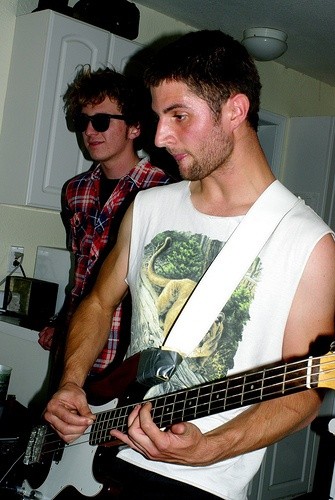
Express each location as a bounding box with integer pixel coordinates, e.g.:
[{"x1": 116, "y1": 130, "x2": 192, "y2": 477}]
[
  {"x1": 246, "y1": 115, "x2": 335, "y2": 500},
  {"x1": 1, "y1": 9, "x2": 287, "y2": 211},
  {"x1": 0, "y1": 315, "x2": 64, "y2": 418}
]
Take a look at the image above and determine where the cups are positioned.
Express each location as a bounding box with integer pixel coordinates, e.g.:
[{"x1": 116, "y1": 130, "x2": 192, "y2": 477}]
[{"x1": 0, "y1": 364, "x2": 13, "y2": 409}]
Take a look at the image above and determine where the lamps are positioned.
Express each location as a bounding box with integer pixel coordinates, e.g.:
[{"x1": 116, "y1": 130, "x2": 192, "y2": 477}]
[{"x1": 241, "y1": 28, "x2": 288, "y2": 62}]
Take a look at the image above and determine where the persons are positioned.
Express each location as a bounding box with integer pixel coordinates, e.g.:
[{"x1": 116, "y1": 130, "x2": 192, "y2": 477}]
[
  {"x1": 37, "y1": 64, "x2": 177, "y2": 399},
  {"x1": 42, "y1": 29, "x2": 334, "y2": 499}
]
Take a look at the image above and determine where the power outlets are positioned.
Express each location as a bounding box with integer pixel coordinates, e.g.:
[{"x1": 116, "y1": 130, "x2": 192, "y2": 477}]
[{"x1": 7, "y1": 246, "x2": 24, "y2": 273}]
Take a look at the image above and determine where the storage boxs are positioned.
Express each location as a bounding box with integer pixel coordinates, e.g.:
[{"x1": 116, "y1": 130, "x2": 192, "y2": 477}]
[{"x1": 34, "y1": 246, "x2": 73, "y2": 316}]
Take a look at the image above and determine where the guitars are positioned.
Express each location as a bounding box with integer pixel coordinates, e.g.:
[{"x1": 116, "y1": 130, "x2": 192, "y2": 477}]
[{"x1": 0, "y1": 349, "x2": 335, "y2": 500}]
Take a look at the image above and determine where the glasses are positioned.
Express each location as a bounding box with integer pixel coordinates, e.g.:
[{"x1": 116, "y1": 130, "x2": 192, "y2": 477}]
[{"x1": 65, "y1": 111, "x2": 123, "y2": 133}]
